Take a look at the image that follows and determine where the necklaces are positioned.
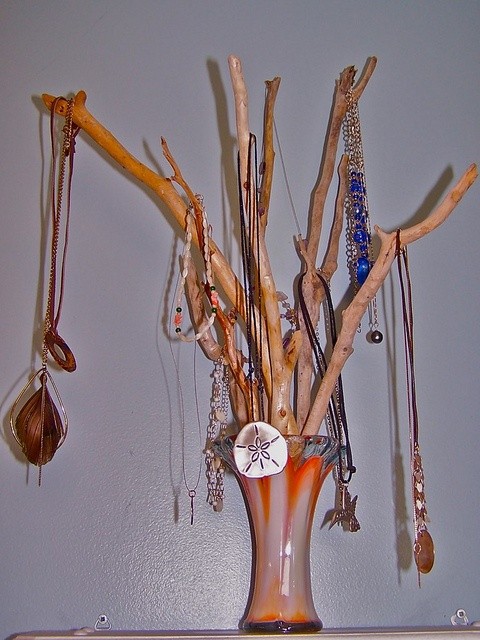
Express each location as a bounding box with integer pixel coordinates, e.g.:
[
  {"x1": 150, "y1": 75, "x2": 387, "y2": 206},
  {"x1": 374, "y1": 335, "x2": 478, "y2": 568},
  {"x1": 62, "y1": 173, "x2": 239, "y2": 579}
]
[
  {"x1": 233, "y1": 133, "x2": 288, "y2": 479},
  {"x1": 299, "y1": 267, "x2": 362, "y2": 533},
  {"x1": 176, "y1": 194, "x2": 217, "y2": 343},
  {"x1": 397, "y1": 229, "x2": 435, "y2": 576},
  {"x1": 9, "y1": 97, "x2": 80, "y2": 486},
  {"x1": 344, "y1": 80, "x2": 386, "y2": 346},
  {"x1": 202, "y1": 357, "x2": 229, "y2": 514},
  {"x1": 169, "y1": 297, "x2": 202, "y2": 525}
]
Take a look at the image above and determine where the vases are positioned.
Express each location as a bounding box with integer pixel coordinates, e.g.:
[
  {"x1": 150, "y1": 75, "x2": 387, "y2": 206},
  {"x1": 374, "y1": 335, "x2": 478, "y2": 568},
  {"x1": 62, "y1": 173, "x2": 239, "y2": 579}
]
[{"x1": 210, "y1": 421, "x2": 339, "y2": 635}]
